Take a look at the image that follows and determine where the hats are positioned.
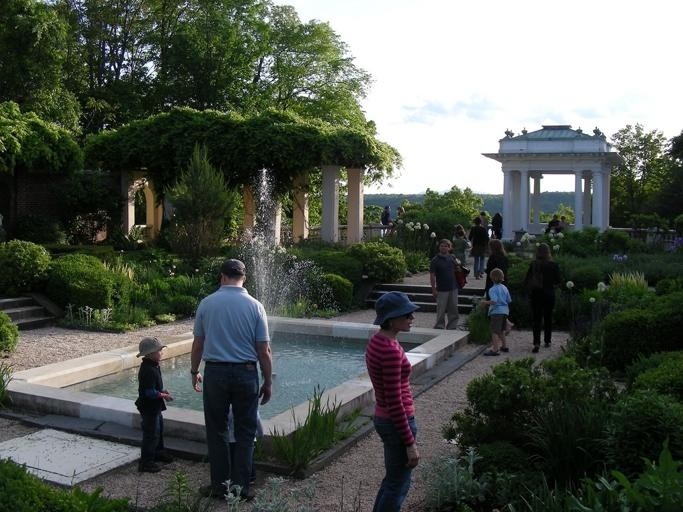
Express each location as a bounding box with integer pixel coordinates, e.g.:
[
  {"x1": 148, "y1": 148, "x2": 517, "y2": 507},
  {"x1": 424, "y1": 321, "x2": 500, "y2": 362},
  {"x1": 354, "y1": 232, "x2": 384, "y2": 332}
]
[
  {"x1": 219, "y1": 260, "x2": 246, "y2": 276},
  {"x1": 136, "y1": 337, "x2": 167, "y2": 358},
  {"x1": 372, "y1": 290, "x2": 420, "y2": 325}
]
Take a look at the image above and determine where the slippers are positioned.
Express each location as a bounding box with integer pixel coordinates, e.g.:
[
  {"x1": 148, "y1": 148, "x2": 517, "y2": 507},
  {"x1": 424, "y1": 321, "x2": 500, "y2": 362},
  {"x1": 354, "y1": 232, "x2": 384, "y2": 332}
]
[
  {"x1": 483, "y1": 350, "x2": 500, "y2": 357},
  {"x1": 500, "y1": 346, "x2": 508, "y2": 352}
]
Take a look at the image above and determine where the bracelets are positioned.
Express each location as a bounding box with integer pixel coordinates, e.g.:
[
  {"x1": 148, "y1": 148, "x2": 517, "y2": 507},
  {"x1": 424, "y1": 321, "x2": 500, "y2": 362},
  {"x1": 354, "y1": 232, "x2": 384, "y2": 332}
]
[{"x1": 191, "y1": 369, "x2": 199, "y2": 375}]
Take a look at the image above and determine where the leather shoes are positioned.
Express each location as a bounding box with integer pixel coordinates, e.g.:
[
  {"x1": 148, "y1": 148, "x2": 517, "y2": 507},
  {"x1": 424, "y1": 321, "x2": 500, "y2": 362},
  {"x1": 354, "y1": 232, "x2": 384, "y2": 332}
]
[
  {"x1": 242, "y1": 483, "x2": 255, "y2": 501},
  {"x1": 545, "y1": 343, "x2": 551, "y2": 348},
  {"x1": 199, "y1": 486, "x2": 226, "y2": 499},
  {"x1": 532, "y1": 344, "x2": 539, "y2": 353}
]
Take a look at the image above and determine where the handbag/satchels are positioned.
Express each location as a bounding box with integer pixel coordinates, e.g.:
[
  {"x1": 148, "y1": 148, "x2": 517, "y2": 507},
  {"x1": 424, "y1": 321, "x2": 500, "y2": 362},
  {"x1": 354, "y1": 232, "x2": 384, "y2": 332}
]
[
  {"x1": 525, "y1": 261, "x2": 543, "y2": 294},
  {"x1": 449, "y1": 254, "x2": 470, "y2": 288}
]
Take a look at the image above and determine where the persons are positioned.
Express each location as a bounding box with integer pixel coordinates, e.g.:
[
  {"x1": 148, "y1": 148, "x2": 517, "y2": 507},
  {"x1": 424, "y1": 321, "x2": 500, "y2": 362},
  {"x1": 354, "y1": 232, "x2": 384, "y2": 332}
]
[
  {"x1": 519, "y1": 241, "x2": 562, "y2": 353},
  {"x1": 557, "y1": 216, "x2": 570, "y2": 232},
  {"x1": 544, "y1": 214, "x2": 560, "y2": 235},
  {"x1": 477, "y1": 268, "x2": 513, "y2": 356},
  {"x1": 133, "y1": 337, "x2": 175, "y2": 474},
  {"x1": 396, "y1": 207, "x2": 406, "y2": 216},
  {"x1": 380, "y1": 206, "x2": 393, "y2": 225},
  {"x1": 426, "y1": 237, "x2": 462, "y2": 330},
  {"x1": 478, "y1": 237, "x2": 516, "y2": 338},
  {"x1": 225, "y1": 401, "x2": 264, "y2": 485},
  {"x1": 448, "y1": 210, "x2": 504, "y2": 285},
  {"x1": 363, "y1": 290, "x2": 420, "y2": 510},
  {"x1": 189, "y1": 257, "x2": 273, "y2": 499}
]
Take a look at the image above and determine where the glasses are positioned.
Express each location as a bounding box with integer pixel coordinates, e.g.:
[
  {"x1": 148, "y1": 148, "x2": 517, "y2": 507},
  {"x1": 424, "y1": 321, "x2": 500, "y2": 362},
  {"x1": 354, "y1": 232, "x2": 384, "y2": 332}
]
[{"x1": 402, "y1": 310, "x2": 417, "y2": 320}]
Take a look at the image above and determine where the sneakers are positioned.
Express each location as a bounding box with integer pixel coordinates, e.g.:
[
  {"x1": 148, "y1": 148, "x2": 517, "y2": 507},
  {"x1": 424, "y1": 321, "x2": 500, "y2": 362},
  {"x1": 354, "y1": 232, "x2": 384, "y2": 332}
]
[{"x1": 138, "y1": 464, "x2": 161, "y2": 473}]
